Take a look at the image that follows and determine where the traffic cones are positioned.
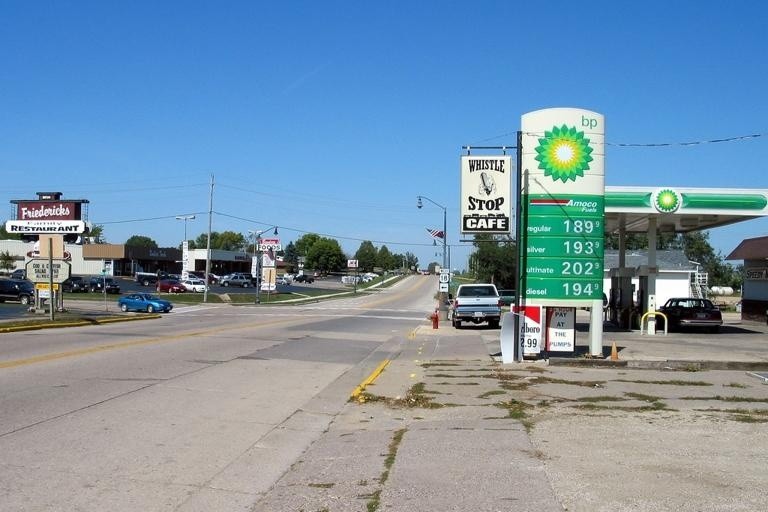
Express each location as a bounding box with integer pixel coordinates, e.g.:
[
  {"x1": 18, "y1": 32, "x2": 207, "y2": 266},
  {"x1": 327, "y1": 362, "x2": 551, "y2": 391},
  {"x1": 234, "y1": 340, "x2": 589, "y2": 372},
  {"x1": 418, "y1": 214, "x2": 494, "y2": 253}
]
[{"x1": 609, "y1": 341, "x2": 620, "y2": 361}]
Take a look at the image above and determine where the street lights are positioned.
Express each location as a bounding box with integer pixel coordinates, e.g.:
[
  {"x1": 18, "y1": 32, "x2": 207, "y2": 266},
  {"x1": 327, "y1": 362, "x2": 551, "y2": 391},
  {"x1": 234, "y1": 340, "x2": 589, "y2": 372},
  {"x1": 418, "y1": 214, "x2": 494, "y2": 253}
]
[
  {"x1": 176, "y1": 214, "x2": 196, "y2": 242},
  {"x1": 253, "y1": 224, "x2": 280, "y2": 305},
  {"x1": 431, "y1": 237, "x2": 451, "y2": 274},
  {"x1": 416, "y1": 194, "x2": 447, "y2": 269},
  {"x1": 246, "y1": 230, "x2": 263, "y2": 257}
]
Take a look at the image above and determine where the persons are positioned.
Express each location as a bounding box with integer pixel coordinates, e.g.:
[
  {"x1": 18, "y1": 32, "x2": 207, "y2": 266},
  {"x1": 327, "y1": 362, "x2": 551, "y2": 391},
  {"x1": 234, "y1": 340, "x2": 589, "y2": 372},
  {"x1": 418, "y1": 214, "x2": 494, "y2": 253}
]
[{"x1": 445, "y1": 294, "x2": 455, "y2": 322}]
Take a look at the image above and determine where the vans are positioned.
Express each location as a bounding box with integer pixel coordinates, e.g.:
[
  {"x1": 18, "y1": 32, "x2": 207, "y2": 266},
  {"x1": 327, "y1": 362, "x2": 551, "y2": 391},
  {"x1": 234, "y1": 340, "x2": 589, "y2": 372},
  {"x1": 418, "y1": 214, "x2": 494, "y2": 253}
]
[{"x1": 496, "y1": 289, "x2": 516, "y2": 305}]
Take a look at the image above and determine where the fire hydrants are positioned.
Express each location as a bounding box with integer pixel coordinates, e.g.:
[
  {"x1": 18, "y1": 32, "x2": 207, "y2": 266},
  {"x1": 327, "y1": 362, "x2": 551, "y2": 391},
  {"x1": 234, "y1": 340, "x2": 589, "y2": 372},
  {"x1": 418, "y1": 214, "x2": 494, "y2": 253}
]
[{"x1": 429, "y1": 310, "x2": 438, "y2": 329}]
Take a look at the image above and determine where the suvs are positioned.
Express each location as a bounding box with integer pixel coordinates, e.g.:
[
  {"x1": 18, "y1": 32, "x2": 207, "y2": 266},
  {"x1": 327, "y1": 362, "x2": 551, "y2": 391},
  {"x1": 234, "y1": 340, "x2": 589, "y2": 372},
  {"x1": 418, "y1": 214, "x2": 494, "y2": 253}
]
[
  {"x1": 90, "y1": 276, "x2": 121, "y2": 294},
  {"x1": 293, "y1": 274, "x2": 314, "y2": 284},
  {"x1": 448, "y1": 282, "x2": 502, "y2": 327},
  {"x1": 0, "y1": 277, "x2": 46, "y2": 305}
]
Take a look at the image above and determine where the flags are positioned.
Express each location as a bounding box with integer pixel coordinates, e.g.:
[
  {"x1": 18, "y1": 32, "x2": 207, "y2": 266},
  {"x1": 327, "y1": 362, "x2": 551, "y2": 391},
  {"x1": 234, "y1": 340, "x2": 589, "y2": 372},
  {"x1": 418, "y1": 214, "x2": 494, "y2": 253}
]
[{"x1": 427, "y1": 228, "x2": 444, "y2": 238}]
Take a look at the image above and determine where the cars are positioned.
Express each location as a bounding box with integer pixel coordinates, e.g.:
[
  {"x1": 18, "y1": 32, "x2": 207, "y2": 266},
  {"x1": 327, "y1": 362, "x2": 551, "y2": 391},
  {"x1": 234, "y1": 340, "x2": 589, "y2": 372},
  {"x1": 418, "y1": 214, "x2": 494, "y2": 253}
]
[
  {"x1": 340, "y1": 272, "x2": 383, "y2": 285},
  {"x1": 136, "y1": 269, "x2": 221, "y2": 293},
  {"x1": 275, "y1": 277, "x2": 291, "y2": 286},
  {"x1": 656, "y1": 297, "x2": 723, "y2": 332},
  {"x1": 387, "y1": 268, "x2": 404, "y2": 275},
  {"x1": 62, "y1": 277, "x2": 89, "y2": 293},
  {"x1": 220, "y1": 271, "x2": 261, "y2": 288},
  {"x1": 9, "y1": 269, "x2": 26, "y2": 280},
  {"x1": 117, "y1": 293, "x2": 173, "y2": 313},
  {"x1": 424, "y1": 271, "x2": 430, "y2": 275}
]
